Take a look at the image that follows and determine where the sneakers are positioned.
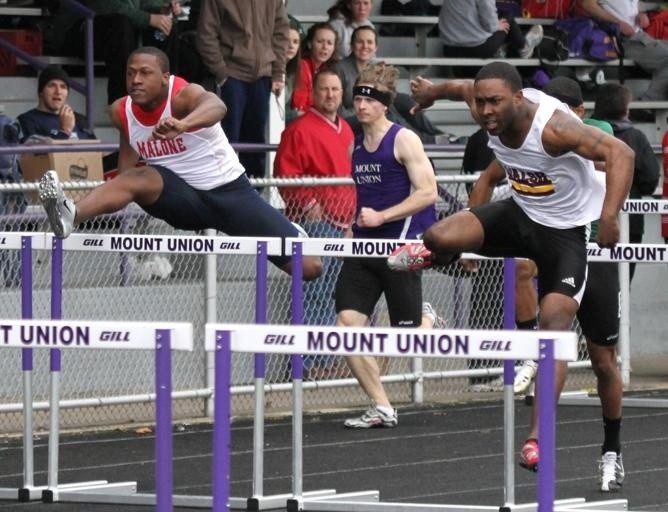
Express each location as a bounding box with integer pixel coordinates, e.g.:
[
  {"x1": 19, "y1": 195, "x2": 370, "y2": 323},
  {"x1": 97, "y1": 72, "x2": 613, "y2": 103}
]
[
  {"x1": 519, "y1": 24, "x2": 543, "y2": 58},
  {"x1": 344, "y1": 405, "x2": 398, "y2": 428},
  {"x1": 519, "y1": 437, "x2": 542, "y2": 470},
  {"x1": 386, "y1": 243, "x2": 434, "y2": 270},
  {"x1": 40, "y1": 170, "x2": 79, "y2": 238},
  {"x1": 422, "y1": 301, "x2": 449, "y2": 329},
  {"x1": 512, "y1": 360, "x2": 536, "y2": 392},
  {"x1": 599, "y1": 451, "x2": 625, "y2": 491}
]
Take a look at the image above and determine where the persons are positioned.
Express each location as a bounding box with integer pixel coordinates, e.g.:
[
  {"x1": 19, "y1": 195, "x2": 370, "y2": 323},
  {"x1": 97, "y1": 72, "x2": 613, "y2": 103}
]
[
  {"x1": 459, "y1": 76, "x2": 627, "y2": 493},
  {"x1": 1, "y1": 2, "x2": 668, "y2": 285},
  {"x1": 387, "y1": 61, "x2": 635, "y2": 473},
  {"x1": 272, "y1": 62, "x2": 354, "y2": 388},
  {"x1": 333, "y1": 61, "x2": 451, "y2": 431}
]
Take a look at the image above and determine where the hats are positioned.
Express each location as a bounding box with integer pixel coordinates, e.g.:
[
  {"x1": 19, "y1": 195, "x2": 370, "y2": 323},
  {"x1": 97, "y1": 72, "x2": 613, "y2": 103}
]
[
  {"x1": 39, "y1": 63, "x2": 70, "y2": 90},
  {"x1": 543, "y1": 76, "x2": 582, "y2": 107}
]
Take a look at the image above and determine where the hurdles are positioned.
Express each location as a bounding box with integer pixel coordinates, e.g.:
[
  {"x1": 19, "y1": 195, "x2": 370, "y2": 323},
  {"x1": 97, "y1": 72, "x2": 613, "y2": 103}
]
[
  {"x1": 1, "y1": 230, "x2": 110, "y2": 502},
  {"x1": 284, "y1": 235, "x2": 585, "y2": 512},
  {"x1": 204, "y1": 322, "x2": 579, "y2": 511},
  {"x1": 557, "y1": 241, "x2": 666, "y2": 511},
  {"x1": 41, "y1": 229, "x2": 337, "y2": 511},
  {"x1": 526, "y1": 198, "x2": 666, "y2": 410}
]
